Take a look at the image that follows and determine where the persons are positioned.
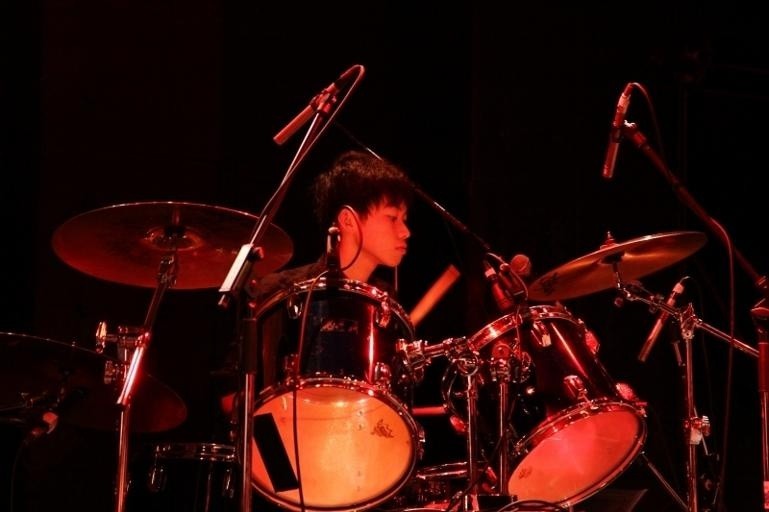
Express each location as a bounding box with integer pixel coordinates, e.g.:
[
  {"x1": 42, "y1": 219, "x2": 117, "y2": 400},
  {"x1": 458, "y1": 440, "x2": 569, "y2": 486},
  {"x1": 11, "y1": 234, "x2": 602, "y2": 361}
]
[{"x1": 208, "y1": 148, "x2": 416, "y2": 415}]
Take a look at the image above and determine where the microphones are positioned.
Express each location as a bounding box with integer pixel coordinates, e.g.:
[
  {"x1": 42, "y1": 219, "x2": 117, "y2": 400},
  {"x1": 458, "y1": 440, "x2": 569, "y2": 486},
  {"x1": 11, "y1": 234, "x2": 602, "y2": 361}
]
[
  {"x1": 482, "y1": 261, "x2": 515, "y2": 312},
  {"x1": 511, "y1": 253, "x2": 535, "y2": 280},
  {"x1": 324, "y1": 226, "x2": 343, "y2": 277},
  {"x1": 638, "y1": 283, "x2": 684, "y2": 363},
  {"x1": 601, "y1": 93, "x2": 631, "y2": 178},
  {"x1": 273, "y1": 75, "x2": 347, "y2": 145}
]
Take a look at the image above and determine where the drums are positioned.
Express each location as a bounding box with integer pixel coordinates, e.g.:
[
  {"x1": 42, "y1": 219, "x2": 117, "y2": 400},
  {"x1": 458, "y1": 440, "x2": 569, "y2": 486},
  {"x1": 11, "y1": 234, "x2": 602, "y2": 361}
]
[
  {"x1": 412, "y1": 338, "x2": 483, "y2": 481},
  {"x1": 228, "y1": 274, "x2": 425, "y2": 507},
  {"x1": 442, "y1": 304, "x2": 649, "y2": 506}
]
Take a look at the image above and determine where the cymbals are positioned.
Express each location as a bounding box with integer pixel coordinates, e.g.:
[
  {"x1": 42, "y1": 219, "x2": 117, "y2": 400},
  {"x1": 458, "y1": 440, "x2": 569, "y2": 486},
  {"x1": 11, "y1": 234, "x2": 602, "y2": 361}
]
[
  {"x1": 526, "y1": 230, "x2": 707, "y2": 300},
  {"x1": 1, "y1": 332, "x2": 186, "y2": 433},
  {"x1": 51, "y1": 200, "x2": 293, "y2": 290}
]
